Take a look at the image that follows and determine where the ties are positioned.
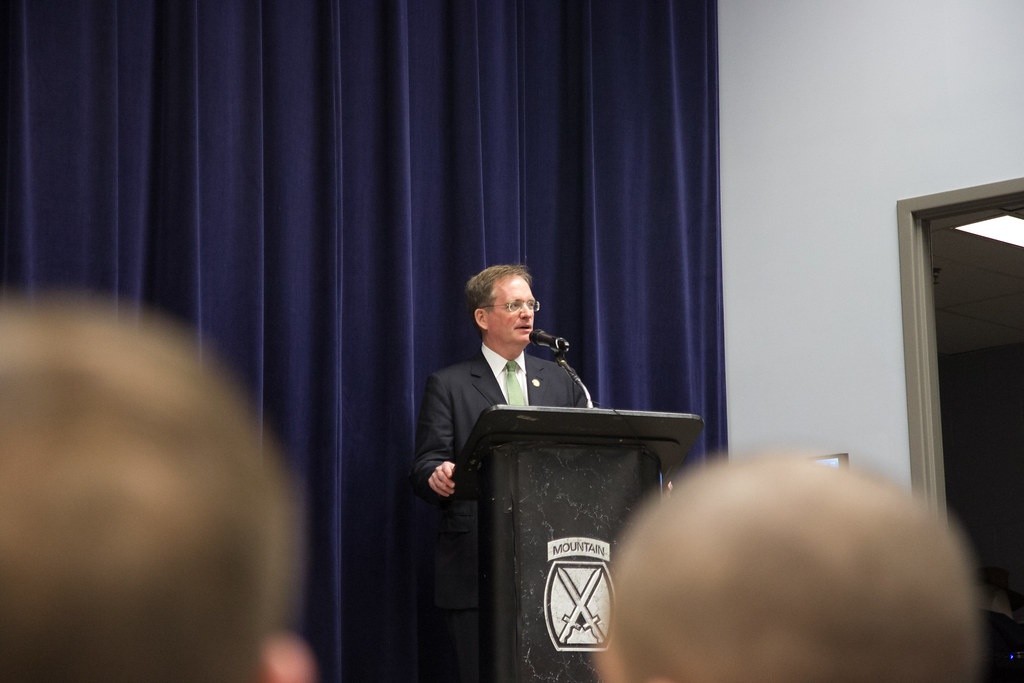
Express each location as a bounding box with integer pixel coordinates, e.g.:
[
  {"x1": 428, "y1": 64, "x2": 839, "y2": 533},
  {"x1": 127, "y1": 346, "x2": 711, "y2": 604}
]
[{"x1": 506, "y1": 361, "x2": 525, "y2": 406}]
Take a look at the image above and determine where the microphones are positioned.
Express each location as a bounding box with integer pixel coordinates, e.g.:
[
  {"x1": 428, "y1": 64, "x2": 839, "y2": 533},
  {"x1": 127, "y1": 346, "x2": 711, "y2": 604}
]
[{"x1": 529, "y1": 329, "x2": 569, "y2": 352}]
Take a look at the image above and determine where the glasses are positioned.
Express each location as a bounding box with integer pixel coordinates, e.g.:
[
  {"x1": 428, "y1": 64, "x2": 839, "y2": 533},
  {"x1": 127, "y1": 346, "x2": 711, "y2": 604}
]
[{"x1": 478, "y1": 299, "x2": 541, "y2": 314}]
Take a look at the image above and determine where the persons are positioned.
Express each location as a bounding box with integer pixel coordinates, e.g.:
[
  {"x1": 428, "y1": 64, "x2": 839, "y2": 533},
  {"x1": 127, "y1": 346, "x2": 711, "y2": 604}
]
[
  {"x1": 410, "y1": 264, "x2": 589, "y2": 683},
  {"x1": 0, "y1": 289, "x2": 321, "y2": 683},
  {"x1": 588, "y1": 447, "x2": 984, "y2": 683}
]
